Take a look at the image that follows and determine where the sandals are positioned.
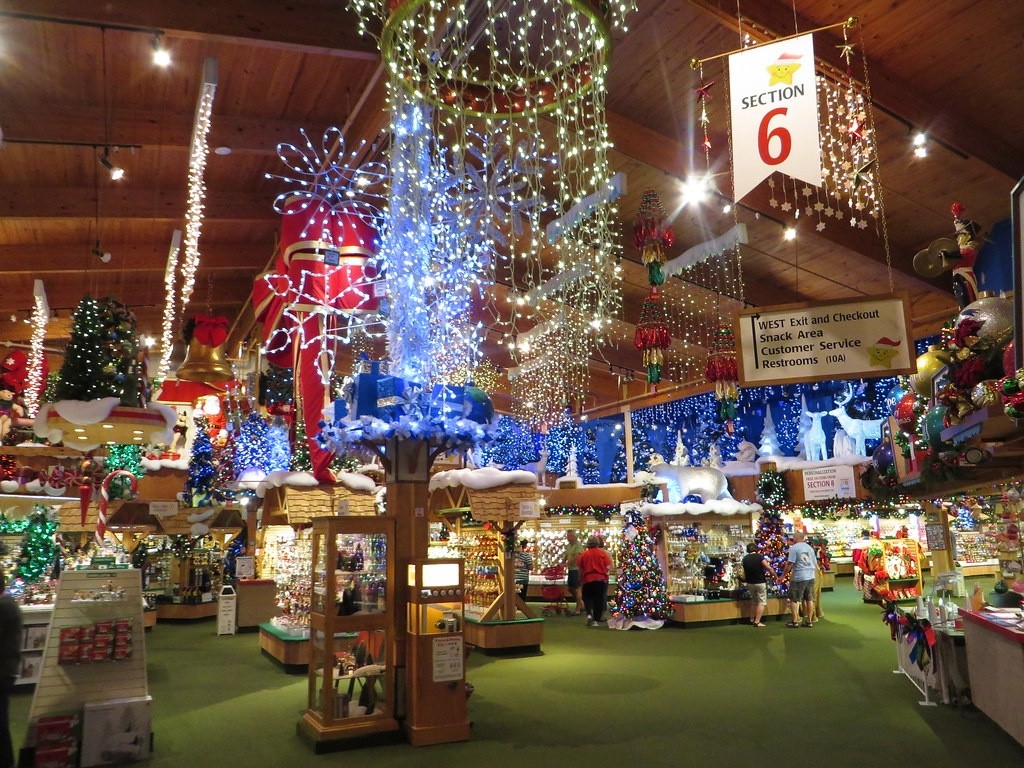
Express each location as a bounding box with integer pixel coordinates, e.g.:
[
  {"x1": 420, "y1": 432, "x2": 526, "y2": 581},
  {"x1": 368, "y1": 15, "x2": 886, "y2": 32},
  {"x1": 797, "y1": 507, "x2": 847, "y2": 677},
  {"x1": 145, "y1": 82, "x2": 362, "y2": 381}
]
[
  {"x1": 786, "y1": 621, "x2": 798, "y2": 628},
  {"x1": 749, "y1": 620, "x2": 766, "y2": 627},
  {"x1": 800, "y1": 621, "x2": 813, "y2": 627}
]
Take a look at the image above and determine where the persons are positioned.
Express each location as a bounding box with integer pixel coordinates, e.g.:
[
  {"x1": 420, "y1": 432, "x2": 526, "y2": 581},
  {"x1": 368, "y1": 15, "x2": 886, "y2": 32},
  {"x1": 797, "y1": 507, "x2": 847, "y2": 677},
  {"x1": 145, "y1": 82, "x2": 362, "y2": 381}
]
[
  {"x1": 851, "y1": 530, "x2": 870, "y2": 591},
  {"x1": 0, "y1": 567, "x2": 23, "y2": 768},
  {"x1": 805, "y1": 559, "x2": 825, "y2": 624},
  {"x1": 577, "y1": 535, "x2": 612, "y2": 627},
  {"x1": 776, "y1": 532, "x2": 818, "y2": 629},
  {"x1": 783, "y1": 537, "x2": 795, "y2": 555},
  {"x1": 515, "y1": 538, "x2": 533, "y2": 611},
  {"x1": 744, "y1": 543, "x2": 779, "y2": 628},
  {"x1": 559, "y1": 530, "x2": 586, "y2": 617},
  {"x1": 590, "y1": 536, "x2": 615, "y2": 623}
]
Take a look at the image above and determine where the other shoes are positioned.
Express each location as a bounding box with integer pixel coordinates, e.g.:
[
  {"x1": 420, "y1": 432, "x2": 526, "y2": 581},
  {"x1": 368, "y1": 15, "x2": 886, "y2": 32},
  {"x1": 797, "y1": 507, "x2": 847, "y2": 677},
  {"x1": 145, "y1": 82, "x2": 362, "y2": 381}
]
[
  {"x1": 807, "y1": 617, "x2": 818, "y2": 623},
  {"x1": 816, "y1": 612, "x2": 824, "y2": 618},
  {"x1": 586, "y1": 615, "x2": 593, "y2": 626},
  {"x1": 797, "y1": 617, "x2": 802, "y2": 622},
  {"x1": 571, "y1": 608, "x2": 586, "y2": 616},
  {"x1": 593, "y1": 620, "x2": 599, "y2": 627}
]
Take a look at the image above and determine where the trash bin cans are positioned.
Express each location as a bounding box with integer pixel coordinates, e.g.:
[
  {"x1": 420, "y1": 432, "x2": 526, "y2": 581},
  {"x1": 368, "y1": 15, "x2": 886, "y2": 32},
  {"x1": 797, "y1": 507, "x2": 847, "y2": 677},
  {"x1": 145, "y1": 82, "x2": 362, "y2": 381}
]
[{"x1": 217, "y1": 585, "x2": 236, "y2": 635}]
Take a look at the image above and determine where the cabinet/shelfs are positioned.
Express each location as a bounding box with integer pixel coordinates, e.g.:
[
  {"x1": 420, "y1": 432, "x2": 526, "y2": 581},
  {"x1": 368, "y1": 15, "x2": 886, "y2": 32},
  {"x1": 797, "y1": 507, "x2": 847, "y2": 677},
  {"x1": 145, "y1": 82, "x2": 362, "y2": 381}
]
[{"x1": 2, "y1": 446, "x2": 1024, "y2": 768}]
